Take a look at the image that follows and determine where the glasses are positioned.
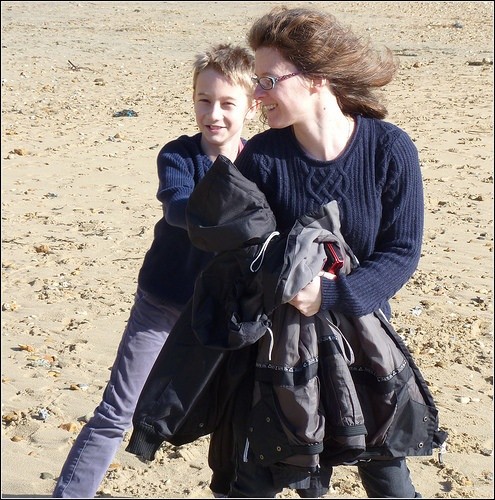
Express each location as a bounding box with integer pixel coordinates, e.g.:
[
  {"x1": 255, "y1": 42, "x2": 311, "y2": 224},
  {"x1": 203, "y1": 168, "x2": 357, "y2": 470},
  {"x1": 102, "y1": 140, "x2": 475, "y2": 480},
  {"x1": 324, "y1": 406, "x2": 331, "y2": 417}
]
[{"x1": 251, "y1": 70, "x2": 301, "y2": 89}]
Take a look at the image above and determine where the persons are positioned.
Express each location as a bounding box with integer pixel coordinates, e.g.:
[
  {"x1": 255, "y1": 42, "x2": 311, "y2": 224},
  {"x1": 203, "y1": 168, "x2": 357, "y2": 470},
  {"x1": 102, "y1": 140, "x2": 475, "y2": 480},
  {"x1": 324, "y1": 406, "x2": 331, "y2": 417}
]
[
  {"x1": 53, "y1": 44, "x2": 257, "y2": 499},
  {"x1": 204, "y1": 9, "x2": 424, "y2": 500}
]
